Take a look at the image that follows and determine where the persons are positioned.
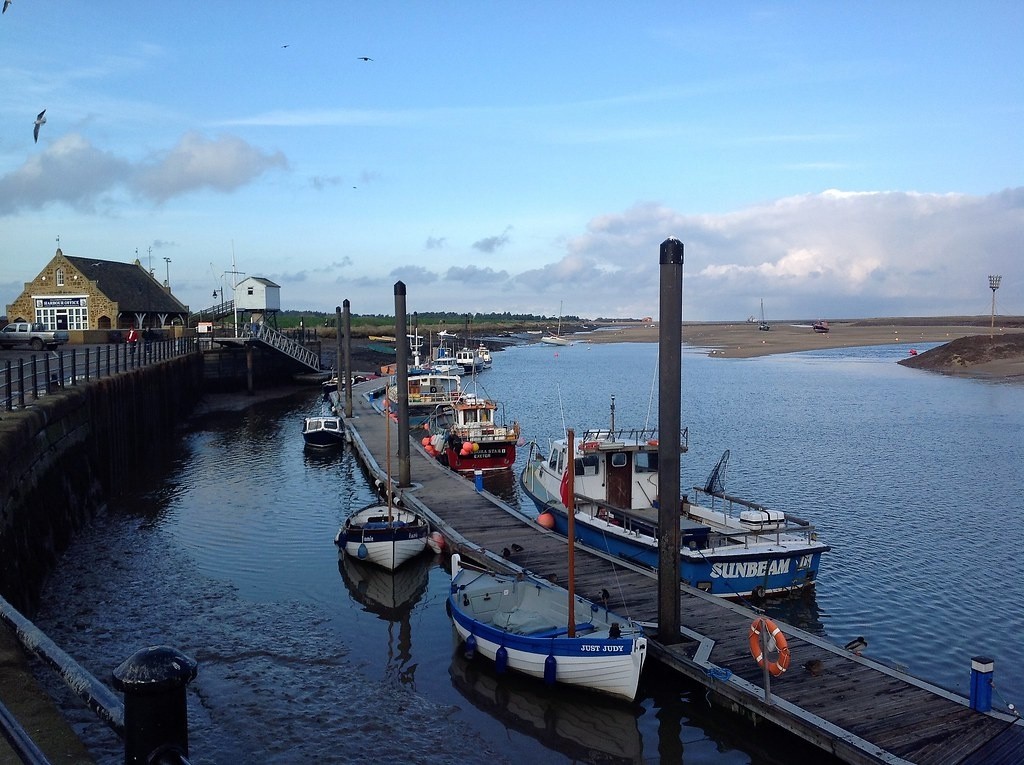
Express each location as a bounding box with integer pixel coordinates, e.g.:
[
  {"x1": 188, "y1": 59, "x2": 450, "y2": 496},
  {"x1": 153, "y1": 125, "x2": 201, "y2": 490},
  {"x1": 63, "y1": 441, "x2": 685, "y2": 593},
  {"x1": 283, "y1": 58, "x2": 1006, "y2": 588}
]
[
  {"x1": 143, "y1": 327, "x2": 154, "y2": 352},
  {"x1": 448, "y1": 430, "x2": 463, "y2": 466},
  {"x1": 126, "y1": 327, "x2": 138, "y2": 354}
]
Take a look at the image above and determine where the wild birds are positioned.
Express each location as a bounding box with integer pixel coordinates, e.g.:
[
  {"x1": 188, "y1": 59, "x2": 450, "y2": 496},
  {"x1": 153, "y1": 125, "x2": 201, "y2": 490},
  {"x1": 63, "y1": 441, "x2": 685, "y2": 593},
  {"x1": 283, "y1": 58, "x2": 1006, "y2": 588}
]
[
  {"x1": 800, "y1": 659, "x2": 824, "y2": 677},
  {"x1": 32, "y1": 109, "x2": 47, "y2": 145},
  {"x1": 844, "y1": 637, "x2": 868, "y2": 656},
  {"x1": 356, "y1": 56, "x2": 375, "y2": 63},
  {"x1": 501, "y1": 543, "x2": 610, "y2": 605},
  {"x1": 279, "y1": 44, "x2": 290, "y2": 49}
]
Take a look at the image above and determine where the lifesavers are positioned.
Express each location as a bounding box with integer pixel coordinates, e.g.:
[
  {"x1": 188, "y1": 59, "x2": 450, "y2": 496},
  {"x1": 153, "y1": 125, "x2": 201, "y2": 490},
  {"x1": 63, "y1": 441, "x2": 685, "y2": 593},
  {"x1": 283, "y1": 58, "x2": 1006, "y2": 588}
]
[
  {"x1": 579, "y1": 441, "x2": 600, "y2": 449},
  {"x1": 750, "y1": 618, "x2": 789, "y2": 676},
  {"x1": 647, "y1": 439, "x2": 658, "y2": 445}
]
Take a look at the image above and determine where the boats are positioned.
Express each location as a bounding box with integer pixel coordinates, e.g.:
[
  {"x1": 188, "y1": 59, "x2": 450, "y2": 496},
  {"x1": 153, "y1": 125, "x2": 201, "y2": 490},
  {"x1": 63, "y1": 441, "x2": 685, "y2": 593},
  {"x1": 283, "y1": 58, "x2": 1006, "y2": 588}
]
[
  {"x1": 444, "y1": 426, "x2": 652, "y2": 702},
  {"x1": 418, "y1": 353, "x2": 524, "y2": 471},
  {"x1": 518, "y1": 392, "x2": 833, "y2": 602},
  {"x1": 383, "y1": 319, "x2": 493, "y2": 428},
  {"x1": 811, "y1": 316, "x2": 830, "y2": 333},
  {"x1": 334, "y1": 380, "x2": 429, "y2": 572},
  {"x1": 301, "y1": 416, "x2": 344, "y2": 448}
]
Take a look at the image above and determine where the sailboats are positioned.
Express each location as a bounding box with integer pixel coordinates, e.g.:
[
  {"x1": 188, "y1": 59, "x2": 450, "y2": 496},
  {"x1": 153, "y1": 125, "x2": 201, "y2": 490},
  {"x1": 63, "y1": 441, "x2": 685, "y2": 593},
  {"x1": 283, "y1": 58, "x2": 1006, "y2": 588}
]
[
  {"x1": 758, "y1": 298, "x2": 771, "y2": 331},
  {"x1": 540, "y1": 301, "x2": 569, "y2": 345}
]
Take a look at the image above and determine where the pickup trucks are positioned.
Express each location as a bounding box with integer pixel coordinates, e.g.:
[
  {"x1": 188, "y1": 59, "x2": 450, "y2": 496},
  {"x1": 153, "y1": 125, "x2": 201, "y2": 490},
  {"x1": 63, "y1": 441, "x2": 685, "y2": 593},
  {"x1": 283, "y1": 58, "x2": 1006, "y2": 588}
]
[{"x1": 0, "y1": 322, "x2": 69, "y2": 352}]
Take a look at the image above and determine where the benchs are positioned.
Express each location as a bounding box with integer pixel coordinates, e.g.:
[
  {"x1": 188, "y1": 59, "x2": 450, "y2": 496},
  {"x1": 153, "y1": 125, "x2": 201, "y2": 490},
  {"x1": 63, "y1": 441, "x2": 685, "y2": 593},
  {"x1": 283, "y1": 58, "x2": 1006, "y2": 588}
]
[
  {"x1": 530, "y1": 621, "x2": 595, "y2": 639},
  {"x1": 355, "y1": 518, "x2": 409, "y2": 528}
]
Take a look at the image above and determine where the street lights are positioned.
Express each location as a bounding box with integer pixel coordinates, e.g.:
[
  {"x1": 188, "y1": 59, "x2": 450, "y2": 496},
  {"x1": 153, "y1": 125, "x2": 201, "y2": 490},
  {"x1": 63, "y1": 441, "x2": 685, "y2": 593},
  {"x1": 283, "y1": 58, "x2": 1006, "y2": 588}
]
[{"x1": 212, "y1": 286, "x2": 224, "y2": 335}]
